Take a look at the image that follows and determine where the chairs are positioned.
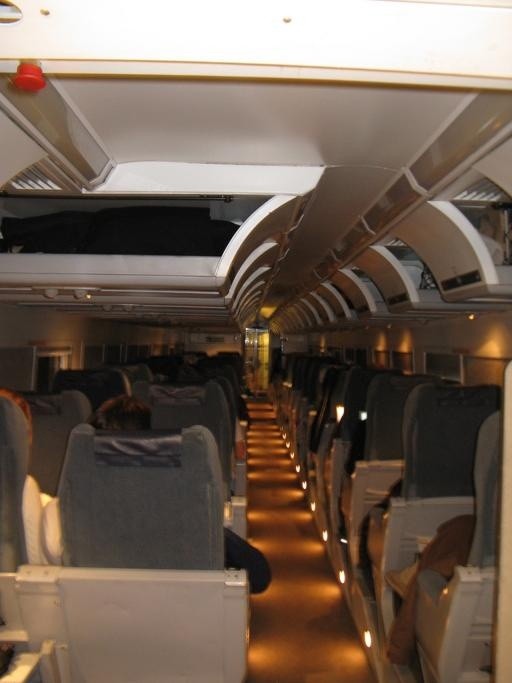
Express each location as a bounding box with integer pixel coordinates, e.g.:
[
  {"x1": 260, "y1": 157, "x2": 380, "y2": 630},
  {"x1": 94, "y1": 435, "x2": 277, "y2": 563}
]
[
  {"x1": 266, "y1": 352, "x2": 503, "y2": 683},
  {"x1": 2, "y1": 348, "x2": 250, "y2": 682}
]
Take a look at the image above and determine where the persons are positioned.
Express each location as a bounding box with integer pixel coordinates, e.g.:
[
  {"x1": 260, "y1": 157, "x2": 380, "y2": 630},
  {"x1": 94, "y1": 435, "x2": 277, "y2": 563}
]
[
  {"x1": 101, "y1": 394, "x2": 270, "y2": 601},
  {"x1": 385, "y1": 514, "x2": 474, "y2": 599},
  {"x1": 235, "y1": 416, "x2": 245, "y2": 462}
]
